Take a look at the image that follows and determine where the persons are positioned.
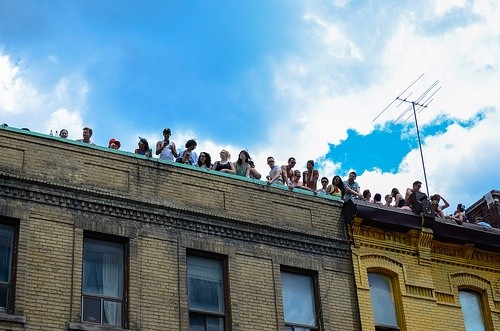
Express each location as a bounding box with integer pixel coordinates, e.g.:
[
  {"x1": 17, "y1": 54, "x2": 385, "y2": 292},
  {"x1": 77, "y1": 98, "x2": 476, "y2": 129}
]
[
  {"x1": 59, "y1": 129, "x2": 68, "y2": 139},
  {"x1": 108, "y1": 139, "x2": 120, "y2": 150},
  {"x1": 156, "y1": 128, "x2": 177, "y2": 161},
  {"x1": 135, "y1": 137, "x2": 152, "y2": 158},
  {"x1": 76, "y1": 127, "x2": 94, "y2": 144},
  {"x1": 475, "y1": 217, "x2": 491, "y2": 229},
  {"x1": 176, "y1": 139, "x2": 469, "y2": 224}
]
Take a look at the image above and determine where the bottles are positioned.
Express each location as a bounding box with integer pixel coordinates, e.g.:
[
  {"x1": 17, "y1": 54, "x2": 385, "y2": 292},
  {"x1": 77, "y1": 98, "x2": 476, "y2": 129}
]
[
  {"x1": 55, "y1": 131, "x2": 59, "y2": 137},
  {"x1": 49, "y1": 130, "x2": 53, "y2": 136},
  {"x1": 149, "y1": 149, "x2": 152, "y2": 157}
]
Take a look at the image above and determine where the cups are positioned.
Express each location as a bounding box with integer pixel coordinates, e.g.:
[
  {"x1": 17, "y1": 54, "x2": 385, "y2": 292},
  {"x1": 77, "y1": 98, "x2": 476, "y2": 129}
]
[{"x1": 334, "y1": 187, "x2": 338, "y2": 193}]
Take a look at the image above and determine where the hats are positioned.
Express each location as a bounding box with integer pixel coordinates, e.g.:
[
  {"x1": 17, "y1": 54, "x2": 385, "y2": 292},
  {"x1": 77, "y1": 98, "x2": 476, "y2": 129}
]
[
  {"x1": 162, "y1": 128, "x2": 171, "y2": 136},
  {"x1": 457, "y1": 203, "x2": 466, "y2": 212},
  {"x1": 138, "y1": 135, "x2": 149, "y2": 150},
  {"x1": 108, "y1": 138, "x2": 121, "y2": 149}
]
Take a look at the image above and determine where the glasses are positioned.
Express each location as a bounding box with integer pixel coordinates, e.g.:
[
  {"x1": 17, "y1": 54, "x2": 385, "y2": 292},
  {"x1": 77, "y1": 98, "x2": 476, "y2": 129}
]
[
  {"x1": 291, "y1": 161, "x2": 296, "y2": 165},
  {"x1": 295, "y1": 174, "x2": 301, "y2": 178},
  {"x1": 432, "y1": 203, "x2": 438, "y2": 206},
  {"x1": 321, "y1": 181, "x2": 327, "y2": 183},
  {"x1": 267, "y1": 160, "x2": 274, "y2": 164},
  {"x1": 219, "y1": 152, "x2": 227, "y2": 155},
  {"x1": 418, "y1": 185, "x2": 421, "y2": 187},
  {"x1": 389, "y1": 197, "x2": 392, "y2": 199},
  {"x1": 199, "y1": 155, "x2": 205, "y2": 157}
]
